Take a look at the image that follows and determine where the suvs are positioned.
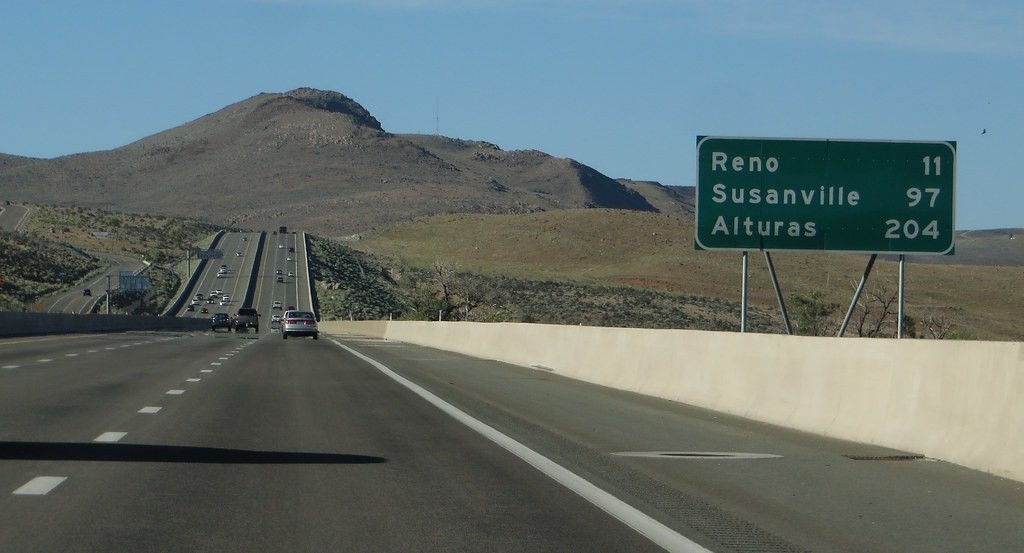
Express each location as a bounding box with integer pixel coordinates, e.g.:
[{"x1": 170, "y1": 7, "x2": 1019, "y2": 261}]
[{"x1": 236, "y1": 308, "x2": 261, "y2": 333}]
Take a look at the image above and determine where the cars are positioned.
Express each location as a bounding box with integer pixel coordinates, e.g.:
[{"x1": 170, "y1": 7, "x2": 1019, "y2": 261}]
[
  {"x1": 272, "y1": 315, "x2": 280, "y2": 325},
  {"x1": 220, "y1": 300, "x2": 227, "y2": 306},
  {"x1": 195, "y1": 294, "x2": 203, "y2": 300},
  {"x1": 212, "y1": 313, "x2": 231, "y2": 331},
  {"x1": 192, "y1": 299, "x2": 199, "y2": 305},
  {"x1": 288, "y1": 272, "x2": 293, "y2": 277},
  {"x1": 207, "y1": 299, "x2": 214, "y2": 303},
  {"x1": 220, "y1": 267, "x2": 227, "y2": 274},
  {"x1": 287, "y1": 257, "x2": 292, "y2": 261},
  {"x1": 276, "y1": 270, "x2": 282, "y2": 275},
  {"x1": 216, "y1": 290, "x2": 222, "y2": 295},
  {"x1": 279, "y1": 244, "x2": 284, "y2": 249},
  {"x1": 273, "y1": 301, "x2": 282, "y2": 310},
  {"x1": 289, "y1": 248, "x2": 294, "y2": 252},
  {"x1": 202, "y1": 308, "x2": 208, "y2": 314},
  {"x1": 223, "y1": 295, "x2": 230, "y2": 301},
  {"x1": 283, "y1": 310, "x2": 318, "y2": 340},
  {"x1": 277, "y1": 277, "x2": 283, "y2": 283},
  {"x1": 217, "y1": 272, "x2": 224, "y2": 278},
  {"x1": 210, "y1": 291, "x2": 217, "y2": 297},
  {"x1": 187, "y1": 305, "x2": 194, "y2": 311},
  {"x1": 83, "y1": 289, "x2": 91, "y2": 296},
  {"x1": 237, "y1": 252, "x2": 242, "y2": 257},
  {"x1": 271, "y1": 327, "x2": 280, "y2": 334}
]
